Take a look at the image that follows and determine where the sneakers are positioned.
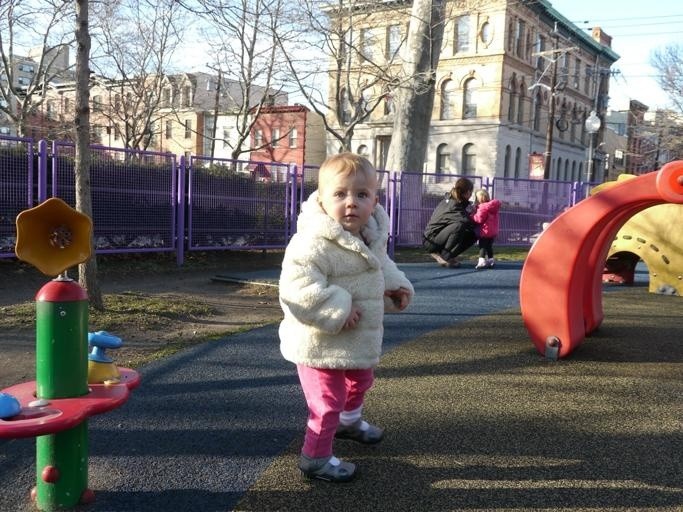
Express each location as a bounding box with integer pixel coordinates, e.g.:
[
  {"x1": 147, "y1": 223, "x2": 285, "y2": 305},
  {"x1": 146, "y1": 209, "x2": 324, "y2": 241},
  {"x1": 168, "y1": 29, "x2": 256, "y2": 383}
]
[
  {"x1": 475, "y1": 259, "x2": 496, "y2": 269},
  {"x1": 299, "y1": 454, "x2": 357, "y2": 483},
  {"x1": 334, "y1": 417, "x2": 385, "y2": 444}
]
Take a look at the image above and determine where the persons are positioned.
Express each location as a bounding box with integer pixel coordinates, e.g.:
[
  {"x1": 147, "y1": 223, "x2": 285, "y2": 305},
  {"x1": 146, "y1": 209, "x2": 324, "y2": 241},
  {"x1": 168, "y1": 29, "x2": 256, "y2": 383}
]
[
  {"x1": 470, "y1": 190, "x2": 502, "y2": 269},
  {"x1": 279, "y1": 153, "x2": 415, "y2": 483},
  {"x1": 423, "y1": 178, "x2": 478, "y2": 268}
]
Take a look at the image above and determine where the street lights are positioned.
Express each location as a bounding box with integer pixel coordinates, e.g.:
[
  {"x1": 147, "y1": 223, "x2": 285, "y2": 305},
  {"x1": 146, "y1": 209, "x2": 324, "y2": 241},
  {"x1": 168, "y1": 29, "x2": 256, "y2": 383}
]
[{"x1": 584, "y1": 111, "x2": 600, "y2": 198}]
